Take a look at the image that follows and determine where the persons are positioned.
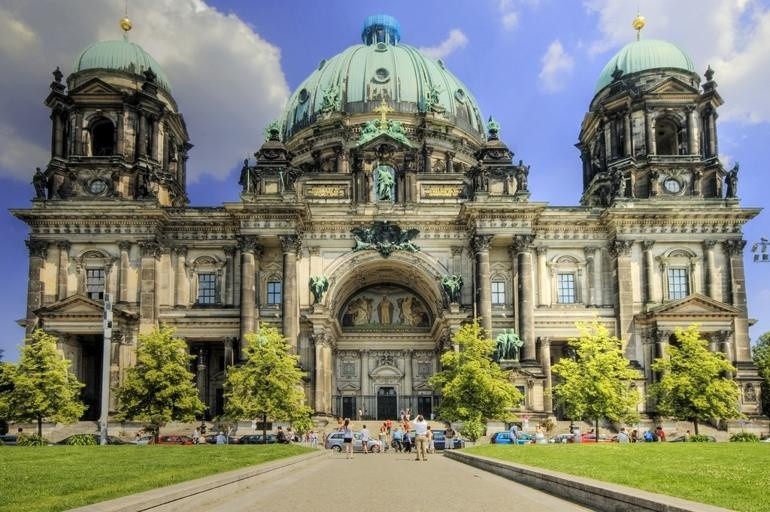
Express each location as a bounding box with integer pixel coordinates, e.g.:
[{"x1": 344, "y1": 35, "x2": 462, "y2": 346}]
[
  {"x1": 527, "y1": 423, "x2": 550, "y2": 444},
  {"x1": 426, "y1": 425, "x2": 435, "y2": 453},
  {"x1": 613, "y1": 426, "x2": 666, "y2": 443},
  {"x1": 412, "y1": 415, "x2": 427, "y2": 462},
  {"x1": 135, "y1": 433, "x2": 141, "y2": 440},
  {"x1": 32, "y1": 167, "x2": 48, "y2": 199},
  {"x1": 147, "y1": 435, "x2": 153, "y2": 445},
  {"x1": 723, "y1": 165, "x2": 740, "y2": 198},
  {"x1": 311, "y1": 274, "x2": 327, "y2": 298},
  {"x1": 378, "y1": 409, "x2": 412, "y2": 454},
  {"x1": 276, "y1": 426, "x2": 294, "y2": 444},
  {"x1": 510, "y1": 424, "x2": 522, "y2": 444},
  {"x1": 194, "y1": 427, "x2": 206, "y2": 444},
  {"x1": 339, "y1": 419, "x2": 354, "y2": 458},
  {"x1": 361, "y1": 424, "x2": 369, "y2": 454},
  {"x1": 444, "y1": 422, "x2": 456, "y2": 449},
  {"x1": 376, "y1": 162, "x2": 397, "y2": 200},
  {"x1": 295, "y1": 431, "x2": 326, "y2": 444},
  {"x1": 555, "y1": 427, "x2": 595, "y2": 443},
  {"x1": 216, "y1": 431, "x2": 227, "y2": 444},
  {"x1": 357, "y1": 409, "x2": 368, "y2": 421},
  {"x1": 443, "y1": 273, "x2": 463, "y2": 298},
  {"x1": 611, "y1": 165, "x2": 628, "y2": 198},
  {"x1": 496, "y1": 327, "x2": 523, "y2": 359},
  {"x1": 685, "y1": 429, "x2": 690, "y2": 442},
  {"x1": 342, "y1": 295, "x2": 429, "y2": 327}
]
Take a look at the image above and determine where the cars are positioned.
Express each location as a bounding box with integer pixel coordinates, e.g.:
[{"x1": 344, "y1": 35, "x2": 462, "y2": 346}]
[
  {"x1": 392, "y1": 430, "x2": 463, "y2": 450},
  {"x1": 1, "y1": 435, "x2": 54, "y2": 446},
  {"x1": 204, "y1": 434, "x2": 239, "y2": 444},
  {"x1": 325, "y1": 431, "x2": 380, "y2": 452},
  {"x1": 567, "y1": 432, "x2": 612, "y2": 442},
  {"x1": 490, "y1": 432, "x2": 532, "y2": 444},
  {"x1": 57, "y1": 434, "x2": 136, "y2": 444},
  {"x1": 135, "y1": 436, "x2": 195, "y2": 445},
  {"x1": 239, "y1": 434, "x2": 280, "y2": 444}
]
